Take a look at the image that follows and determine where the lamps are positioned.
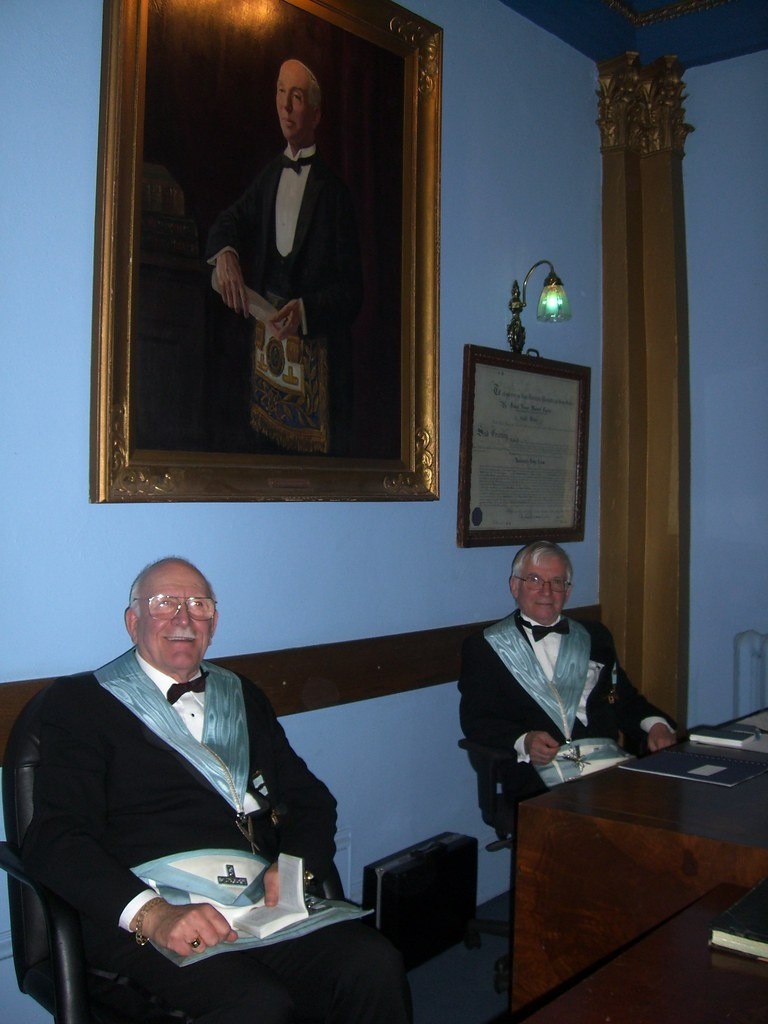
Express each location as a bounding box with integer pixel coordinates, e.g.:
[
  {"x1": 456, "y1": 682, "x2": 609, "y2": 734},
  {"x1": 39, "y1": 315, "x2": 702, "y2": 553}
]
[{"x1": 509, "y1": 260, "x2": 571, "y2": 348}]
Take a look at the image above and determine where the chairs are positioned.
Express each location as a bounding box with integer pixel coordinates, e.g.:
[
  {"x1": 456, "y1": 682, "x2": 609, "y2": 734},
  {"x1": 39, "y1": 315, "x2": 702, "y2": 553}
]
[
  {"x1": 457, "y1": 651, "x2": 515, "y2": 850},
  {"x1": 0, "y1": 684, "x2": 344, "y2": 1024}
]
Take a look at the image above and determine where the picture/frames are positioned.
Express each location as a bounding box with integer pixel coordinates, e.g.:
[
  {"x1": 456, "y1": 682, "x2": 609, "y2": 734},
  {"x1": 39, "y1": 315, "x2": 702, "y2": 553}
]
[{"x1": 88, "y1": 0, "x2": 443, "y2": 503}]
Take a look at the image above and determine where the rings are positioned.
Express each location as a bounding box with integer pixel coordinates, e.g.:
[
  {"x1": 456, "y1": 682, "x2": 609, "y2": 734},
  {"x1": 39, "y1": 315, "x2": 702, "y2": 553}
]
[{"x1": 190, "y1": 937, "x2": 200, "y2": 948}]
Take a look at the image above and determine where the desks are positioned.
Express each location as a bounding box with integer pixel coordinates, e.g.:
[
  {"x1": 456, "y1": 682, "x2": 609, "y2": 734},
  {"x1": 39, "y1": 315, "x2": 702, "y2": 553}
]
[
  {"x1": 509, "y1": 709, "x2": 768, "y2": 1017},
  {"x1": 521, "y1": 881, "x2": 768, "y2": 1024}
]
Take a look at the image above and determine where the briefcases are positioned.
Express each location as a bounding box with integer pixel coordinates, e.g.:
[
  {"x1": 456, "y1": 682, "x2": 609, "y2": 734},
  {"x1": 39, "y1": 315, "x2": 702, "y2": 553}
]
[{"x1": 362, "y1": 831, "x2": 478, "y2": 972}]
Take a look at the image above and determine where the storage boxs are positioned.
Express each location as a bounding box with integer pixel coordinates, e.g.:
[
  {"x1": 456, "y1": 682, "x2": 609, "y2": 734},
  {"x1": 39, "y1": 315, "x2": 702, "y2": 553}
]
[{"x1": 362, "y1": 832, "x2": 479, "y2": 973}]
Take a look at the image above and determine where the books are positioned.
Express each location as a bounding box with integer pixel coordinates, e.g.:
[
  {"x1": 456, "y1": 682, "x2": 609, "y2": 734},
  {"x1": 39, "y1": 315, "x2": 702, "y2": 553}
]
[
  {"x1": 618, "y1": 750, "x2": 768, "y2": 787},
  {"x1": 689, "y1": 728, "x2": 755, "y2": 746},
  {"x1": 708, "y1": 876, "x2": 768, "y2": 963},
  {"x1": 232, "y1": 853, "x2": 309, "y2": 940}
]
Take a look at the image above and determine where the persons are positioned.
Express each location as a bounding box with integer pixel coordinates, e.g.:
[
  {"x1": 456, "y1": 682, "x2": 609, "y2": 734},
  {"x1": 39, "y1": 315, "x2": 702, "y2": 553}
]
[
  {"x1": 460, "y1": 541, "x2": 677, "y2": 831},
  {"x1": 21, "y1": 558, "x2": 412, "y2": 1023}
]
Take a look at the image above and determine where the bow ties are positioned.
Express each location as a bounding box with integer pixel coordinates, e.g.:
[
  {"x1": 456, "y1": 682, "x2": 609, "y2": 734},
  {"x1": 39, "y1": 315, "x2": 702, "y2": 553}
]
[
  {"x1": 519, "y1": 616, "x2": 570, "y2": 642},
  {"x1": 167, "y1": 671, "x2": 210, "y2": 705}
]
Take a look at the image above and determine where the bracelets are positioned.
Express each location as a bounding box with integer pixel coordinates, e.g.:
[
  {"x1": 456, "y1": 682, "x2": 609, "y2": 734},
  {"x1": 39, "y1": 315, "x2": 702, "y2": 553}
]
[{"x1": 137, "y1": 897, "x2": 165, "y2": 945}]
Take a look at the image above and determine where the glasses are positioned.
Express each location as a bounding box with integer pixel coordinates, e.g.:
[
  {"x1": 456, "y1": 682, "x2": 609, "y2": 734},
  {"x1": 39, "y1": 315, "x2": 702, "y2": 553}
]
[
  {"x1": 133, "y1": 595, "x2": 217, "y2": 620},
  {"x1": 515, "y1": 575, "x2": 572, "y2": 592}
]
